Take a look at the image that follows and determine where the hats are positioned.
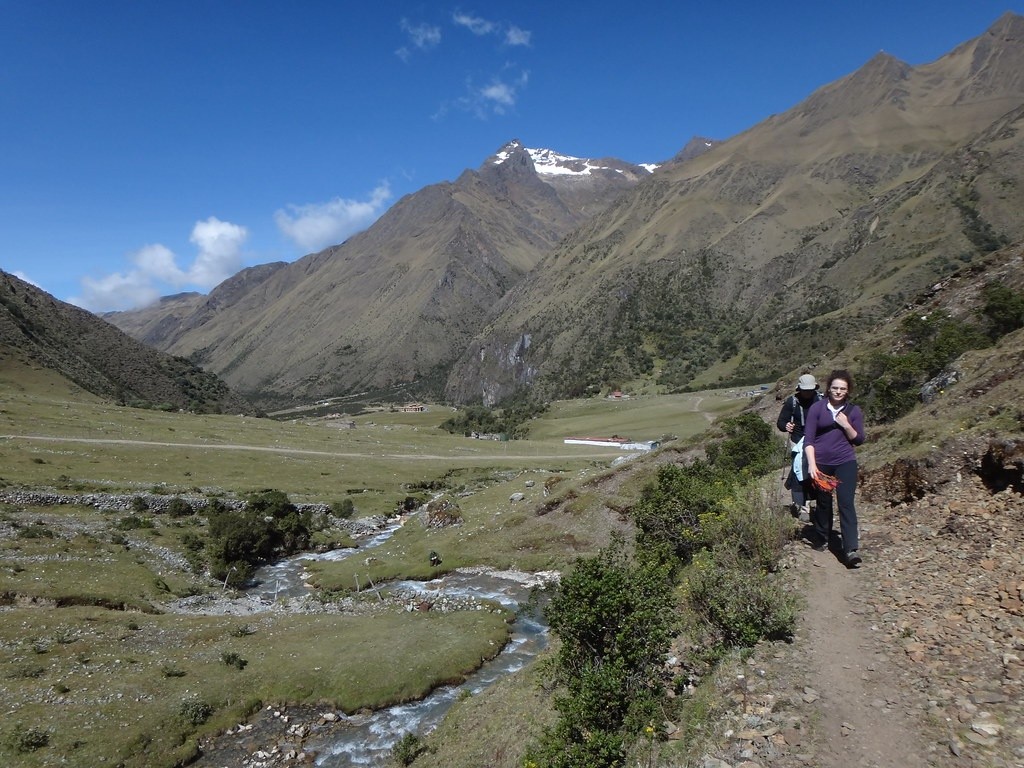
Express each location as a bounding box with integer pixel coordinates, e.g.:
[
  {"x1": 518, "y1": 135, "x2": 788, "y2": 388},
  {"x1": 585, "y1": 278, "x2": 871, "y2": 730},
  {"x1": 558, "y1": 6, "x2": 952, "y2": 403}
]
[{"x1": 795, "y1": 374, "x2": 819, "y2": 391}]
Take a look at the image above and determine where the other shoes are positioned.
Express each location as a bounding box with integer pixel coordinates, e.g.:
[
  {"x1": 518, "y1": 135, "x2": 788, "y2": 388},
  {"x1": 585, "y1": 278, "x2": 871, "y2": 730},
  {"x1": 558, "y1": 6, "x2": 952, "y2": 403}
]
[
  {"x1": 798, "y1": 511, "x2": 810, "y2": 524},
  {"x1": 845, "y1": 551, "x2": 862, "y2": 567},
  {"x1": 818, "y1": 537, "x2": 829, "y2": 551}
]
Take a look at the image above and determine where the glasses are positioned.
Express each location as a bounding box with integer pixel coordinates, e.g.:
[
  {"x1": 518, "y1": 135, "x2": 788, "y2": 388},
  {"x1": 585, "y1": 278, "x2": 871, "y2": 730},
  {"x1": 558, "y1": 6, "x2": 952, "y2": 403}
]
[{"x1": 828, "y1": 387, "x2": 849, "y2": 395}]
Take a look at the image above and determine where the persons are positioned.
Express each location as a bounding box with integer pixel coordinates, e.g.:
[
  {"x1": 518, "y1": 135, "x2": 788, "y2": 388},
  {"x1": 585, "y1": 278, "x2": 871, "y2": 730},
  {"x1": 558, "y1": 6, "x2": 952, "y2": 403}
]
[
  {"x1": 804, "y1": 370, "x2": 864, "y2": 567},
  {"x1": 777, "y1": 374, "x2": 820, "y2": 522}
]
